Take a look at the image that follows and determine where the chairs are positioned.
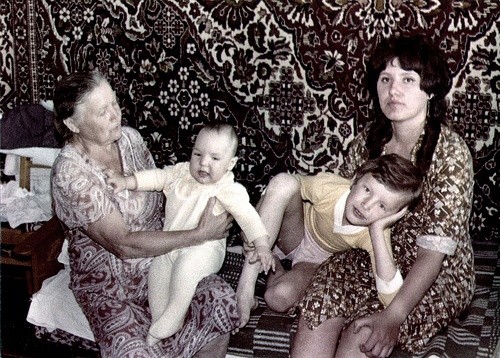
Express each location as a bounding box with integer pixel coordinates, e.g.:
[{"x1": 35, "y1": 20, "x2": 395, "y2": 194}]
[{"x1": 0, "y1": 104, "x2": 69, "y2": 295}]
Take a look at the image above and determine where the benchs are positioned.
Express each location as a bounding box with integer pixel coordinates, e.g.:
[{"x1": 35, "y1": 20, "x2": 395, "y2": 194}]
[{"x1": 27, "y1": 216, "x2": 500, "y2": 358}]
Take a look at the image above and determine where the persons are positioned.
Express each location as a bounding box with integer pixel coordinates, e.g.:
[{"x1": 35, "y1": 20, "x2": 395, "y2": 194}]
[
  {"x1": 230, "y1": 153, "x2": 426, "y2": 336},
  {"x1": 102, "y1": 119, "x2": 276, "y2": 346},
  {"x1": 287, "y1": 31, "x2": 475, "y2": 358},
  {"x1": 50, "y1": 70, "x2": 242, "y2": 358}
]
